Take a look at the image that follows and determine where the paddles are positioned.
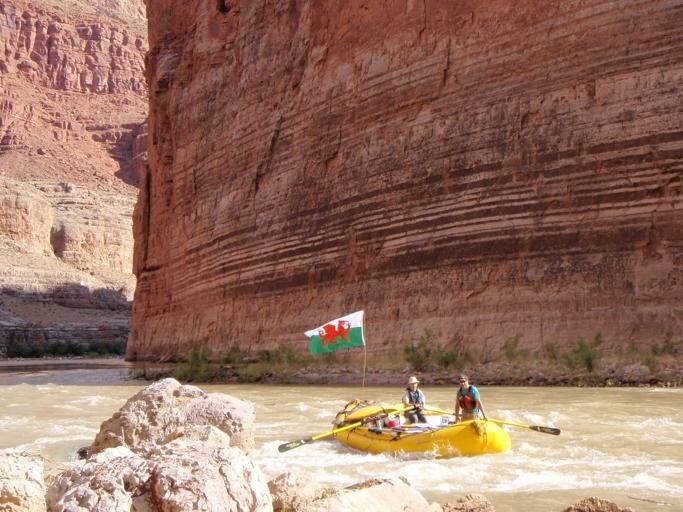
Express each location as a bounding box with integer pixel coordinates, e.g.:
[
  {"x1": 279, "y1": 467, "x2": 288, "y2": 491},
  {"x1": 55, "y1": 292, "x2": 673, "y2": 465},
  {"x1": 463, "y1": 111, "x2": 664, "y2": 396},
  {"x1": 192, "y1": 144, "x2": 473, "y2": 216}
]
[
  {"x1": 424, "y1": 409, "x2": 561, "y2": 435},
  {"x1": 279, "y1": 403, "x2": 416, "y2": 453}
]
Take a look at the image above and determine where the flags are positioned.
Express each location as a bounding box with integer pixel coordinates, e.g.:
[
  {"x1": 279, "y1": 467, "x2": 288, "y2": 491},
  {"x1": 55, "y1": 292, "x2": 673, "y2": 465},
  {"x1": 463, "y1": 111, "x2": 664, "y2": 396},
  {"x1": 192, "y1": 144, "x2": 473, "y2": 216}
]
[{"x1": 303, "y1": 309, "x2": 366, "y2": 355}]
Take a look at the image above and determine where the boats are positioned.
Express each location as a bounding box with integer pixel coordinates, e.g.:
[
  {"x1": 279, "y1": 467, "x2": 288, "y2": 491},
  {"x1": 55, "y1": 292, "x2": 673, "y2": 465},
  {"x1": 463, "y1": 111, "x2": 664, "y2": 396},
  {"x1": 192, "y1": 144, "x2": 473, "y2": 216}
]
[{"x1": 333, "y1": 397, "x2": 510, "y2": 462}]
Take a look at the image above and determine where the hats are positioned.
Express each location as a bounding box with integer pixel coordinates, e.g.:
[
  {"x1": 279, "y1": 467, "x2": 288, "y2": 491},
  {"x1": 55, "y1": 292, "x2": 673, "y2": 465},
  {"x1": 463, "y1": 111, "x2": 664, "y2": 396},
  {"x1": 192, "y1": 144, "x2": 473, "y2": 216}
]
[
  {"x1": 458, "y1": 375, "x2": 467, "y2": 382},
  {"x1": 407, "y1": 376, "x2": 420, "y2": 384}
]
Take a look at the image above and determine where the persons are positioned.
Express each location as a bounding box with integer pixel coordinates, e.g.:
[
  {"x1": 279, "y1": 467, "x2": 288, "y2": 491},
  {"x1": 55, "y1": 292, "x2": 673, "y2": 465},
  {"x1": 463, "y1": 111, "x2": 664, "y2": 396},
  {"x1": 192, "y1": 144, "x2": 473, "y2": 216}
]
[
  {"x1": 402, "y1": 376, "x2": 428, "y2": 424},
  {"x1": 454, "y1": 374, "x2": 488, "y2": 424}
]
